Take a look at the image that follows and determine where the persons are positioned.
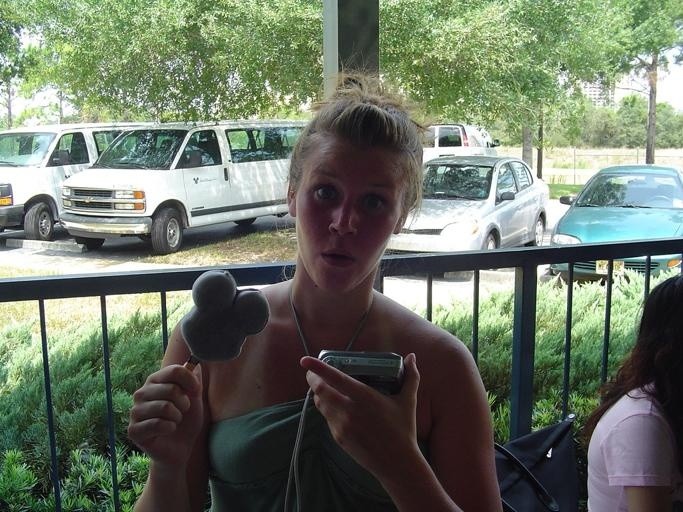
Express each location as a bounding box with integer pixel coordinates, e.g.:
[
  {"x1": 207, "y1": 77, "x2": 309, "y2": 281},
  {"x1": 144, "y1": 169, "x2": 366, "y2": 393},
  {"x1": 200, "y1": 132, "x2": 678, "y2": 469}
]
[
  {"x1": 122, "y1": 50, "x2": 501, "y2": 511},
  {"x1": 569, "y1": 275, "x2": 682, "y2": 508}
]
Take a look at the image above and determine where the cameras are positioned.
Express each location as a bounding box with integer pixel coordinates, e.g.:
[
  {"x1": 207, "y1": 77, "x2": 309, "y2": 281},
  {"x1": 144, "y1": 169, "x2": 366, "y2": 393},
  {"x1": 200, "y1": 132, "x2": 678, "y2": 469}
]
[{"x1": 318, "y1": 349, "x2": 404, "y2": 395}]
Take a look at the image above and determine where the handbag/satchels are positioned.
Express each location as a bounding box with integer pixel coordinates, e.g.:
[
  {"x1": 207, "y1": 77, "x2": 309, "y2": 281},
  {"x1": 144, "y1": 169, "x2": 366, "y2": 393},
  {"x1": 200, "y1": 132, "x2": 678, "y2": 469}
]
[{"x1": 494, "y1": 412, "x2": 582, "y2": 507}]
